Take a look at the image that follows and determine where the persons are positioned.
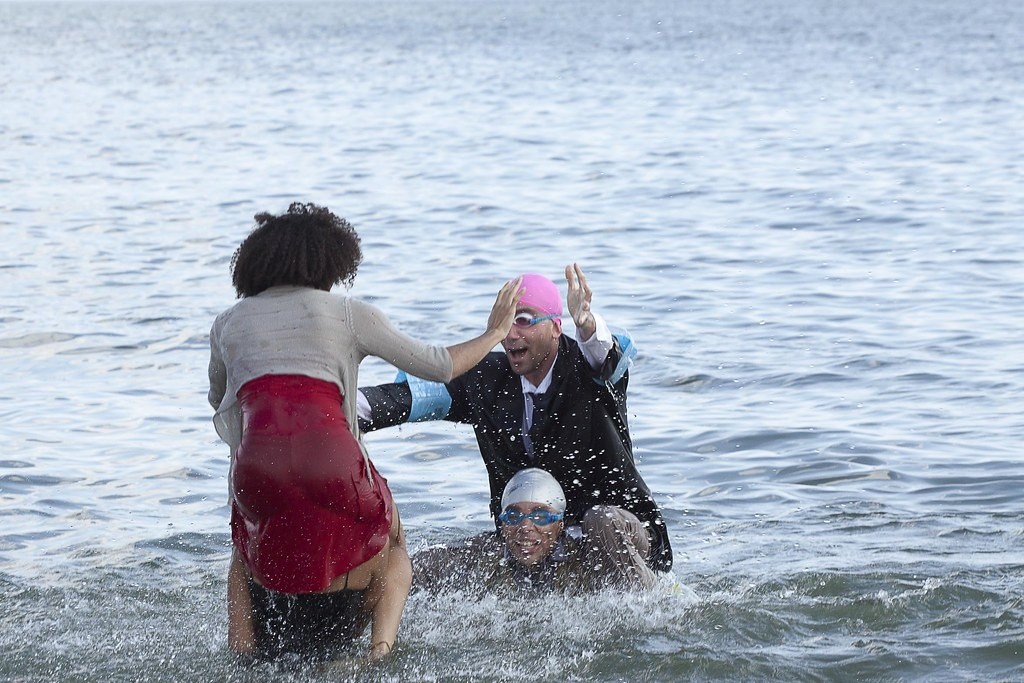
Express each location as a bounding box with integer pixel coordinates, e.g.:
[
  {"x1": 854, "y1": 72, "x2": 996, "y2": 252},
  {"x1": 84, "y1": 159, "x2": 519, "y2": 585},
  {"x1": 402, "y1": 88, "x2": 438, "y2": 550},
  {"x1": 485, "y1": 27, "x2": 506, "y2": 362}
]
[
  {"x1": 357, "y1": 263, "x2": 673, "y2": 602},
  {"x1": 208, "y1": 202, "x2": 525, "y2": 658}
]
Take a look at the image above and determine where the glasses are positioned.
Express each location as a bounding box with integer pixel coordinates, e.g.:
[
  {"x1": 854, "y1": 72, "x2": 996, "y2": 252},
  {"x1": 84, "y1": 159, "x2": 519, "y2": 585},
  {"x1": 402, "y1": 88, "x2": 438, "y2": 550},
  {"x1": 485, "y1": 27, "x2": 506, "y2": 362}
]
[
  {"x1": 512, "y1": 312, "x2": 561, "y2": 328},
  {"x1": 498, "y1": 507, "x2": 564, "y2": 525}
]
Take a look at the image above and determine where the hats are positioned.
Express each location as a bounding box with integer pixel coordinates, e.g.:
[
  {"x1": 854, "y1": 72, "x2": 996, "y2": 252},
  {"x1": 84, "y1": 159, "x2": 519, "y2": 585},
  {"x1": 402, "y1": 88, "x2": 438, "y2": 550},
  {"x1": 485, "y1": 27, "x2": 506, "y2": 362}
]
[
  {"x1": 501, "y1": 468, "x2": 566, "y2": 515},
  {"x1": 510, "y1": 273, "x2": 562, "y2": 325}
]
